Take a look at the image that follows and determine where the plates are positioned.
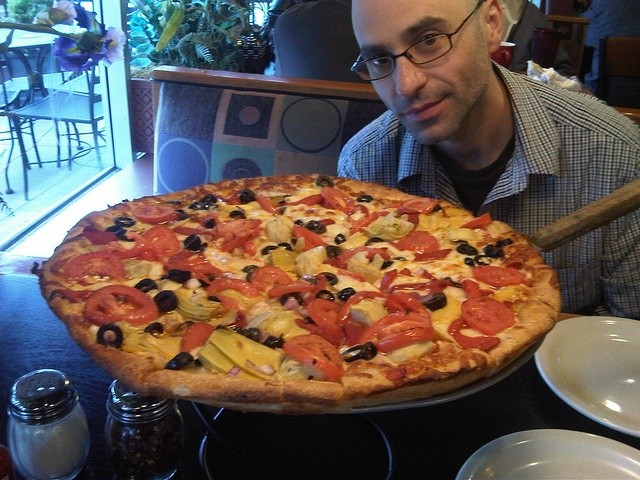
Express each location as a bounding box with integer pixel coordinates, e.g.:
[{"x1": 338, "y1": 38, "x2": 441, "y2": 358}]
[
  {"x1": 455, "y1": 430, "x2": 640, "y2": 480},
  {"x1": 534, "y1": 315, "x2": 640, "y2": 438}
]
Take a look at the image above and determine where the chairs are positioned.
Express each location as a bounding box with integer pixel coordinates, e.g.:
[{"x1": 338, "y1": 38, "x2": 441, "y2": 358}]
[
  {"x1": 598, "y1": 35, "x2": 640, "y2": 103},
  {"x1": 544, "y1": 15, "x2": 590, "y2": 75},
  {"x1": 0, "y1": 42, "x2": 105, "y2": 200}
]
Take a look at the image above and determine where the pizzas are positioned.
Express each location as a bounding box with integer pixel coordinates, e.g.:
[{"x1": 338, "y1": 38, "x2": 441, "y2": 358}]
[{"x1": 36, "y1": 172, "x2": 561, "y2": 407}]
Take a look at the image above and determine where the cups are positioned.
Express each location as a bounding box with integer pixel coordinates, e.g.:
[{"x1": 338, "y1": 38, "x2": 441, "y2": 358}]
[
  {"x1": 531, "y1": 28, "x2": 562, "y2": 68},
  {"x1": 491, "y1": 41, "x2": 516, "y2": 69}
]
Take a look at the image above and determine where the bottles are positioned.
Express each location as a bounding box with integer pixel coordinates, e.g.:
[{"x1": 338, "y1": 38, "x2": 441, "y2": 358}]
[
  {"x1": 104, "y1": 378, "x2": 182, "y2": 480},
  {"x1": 5, "y1": 370, "x2": 88, "y2": 480}
]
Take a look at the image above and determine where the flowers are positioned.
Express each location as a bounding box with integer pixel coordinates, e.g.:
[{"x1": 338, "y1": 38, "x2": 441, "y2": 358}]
[{"x1": 37, "y1": 0, "x2": 126, "y2": 72}]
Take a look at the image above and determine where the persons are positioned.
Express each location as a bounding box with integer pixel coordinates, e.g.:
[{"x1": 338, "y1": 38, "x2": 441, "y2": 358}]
[
  {"x1": 273, "y1": 0, "x2": 371, "y2": 83},
  {"x1": 500, "y1": 0, "x2": 553, "y2": 71},
  {"x1": 338, "y1": 0, "x2": 640, "y2": 319},
  {"x1": 583, "y1": 0, "x2": 640, "y2": 92}
]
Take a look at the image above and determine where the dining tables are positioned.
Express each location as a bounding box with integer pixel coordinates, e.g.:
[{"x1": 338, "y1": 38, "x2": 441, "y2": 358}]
[{"x1": 0, "y1": 272, "x2": 640, "y2": 480}]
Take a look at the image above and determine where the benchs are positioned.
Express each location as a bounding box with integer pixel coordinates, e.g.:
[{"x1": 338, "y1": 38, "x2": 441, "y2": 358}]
[{"x1": 146, "y1": 67, "x2": 386, "y2": 199}]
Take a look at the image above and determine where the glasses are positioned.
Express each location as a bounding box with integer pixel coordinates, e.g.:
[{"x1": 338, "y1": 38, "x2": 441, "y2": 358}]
[{"x1": 350, "y1": 0, "x2": 489, "y2": 82}]
